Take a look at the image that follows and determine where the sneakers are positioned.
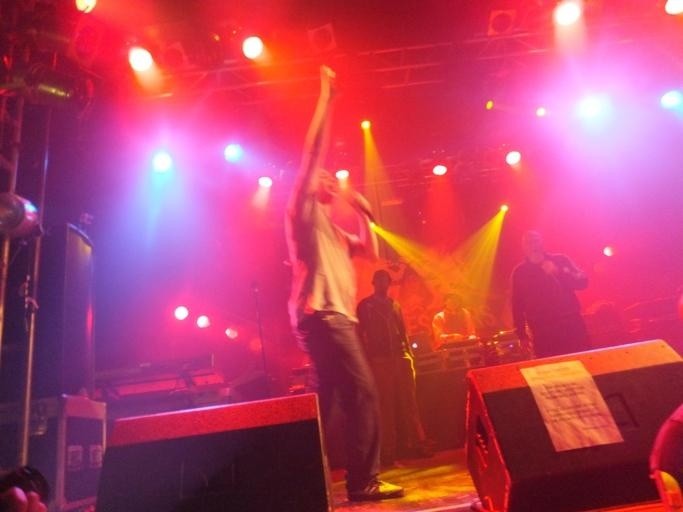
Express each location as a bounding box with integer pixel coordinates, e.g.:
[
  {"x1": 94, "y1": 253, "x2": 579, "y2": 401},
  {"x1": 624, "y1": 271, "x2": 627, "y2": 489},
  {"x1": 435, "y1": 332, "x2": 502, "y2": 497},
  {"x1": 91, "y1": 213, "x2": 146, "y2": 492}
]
[{"x1": 346, "y1": 478, "x2": 404, "y2": 501}]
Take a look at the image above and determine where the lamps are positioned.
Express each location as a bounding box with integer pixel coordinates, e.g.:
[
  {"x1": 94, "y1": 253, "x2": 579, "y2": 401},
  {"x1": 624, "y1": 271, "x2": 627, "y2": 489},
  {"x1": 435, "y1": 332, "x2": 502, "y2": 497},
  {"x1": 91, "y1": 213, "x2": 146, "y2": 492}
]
[{"x1": 3, "y1": 66, "x2": 99, "y2": 117}]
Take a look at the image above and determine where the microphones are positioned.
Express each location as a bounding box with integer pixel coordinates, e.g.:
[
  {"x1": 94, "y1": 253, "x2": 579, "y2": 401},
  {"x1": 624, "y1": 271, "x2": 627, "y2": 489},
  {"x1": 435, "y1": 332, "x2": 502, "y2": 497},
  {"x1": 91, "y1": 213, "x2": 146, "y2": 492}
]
[{"x1": 356, "y1": 203, "x2": 374, "y2": 225}]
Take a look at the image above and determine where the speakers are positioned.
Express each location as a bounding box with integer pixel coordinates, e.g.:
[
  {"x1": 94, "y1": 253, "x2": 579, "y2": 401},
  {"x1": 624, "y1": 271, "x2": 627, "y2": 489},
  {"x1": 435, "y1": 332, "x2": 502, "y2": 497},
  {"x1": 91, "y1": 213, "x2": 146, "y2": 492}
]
[
  {"x1": 94, "y1": 393, "x2": 332, "y2": 512},
  {"x1": 463, "y1": 339, "x2": 683, "y2": 512},
  {"x1": 0, "y1": 222, "x2": 97, "y2": 402}
]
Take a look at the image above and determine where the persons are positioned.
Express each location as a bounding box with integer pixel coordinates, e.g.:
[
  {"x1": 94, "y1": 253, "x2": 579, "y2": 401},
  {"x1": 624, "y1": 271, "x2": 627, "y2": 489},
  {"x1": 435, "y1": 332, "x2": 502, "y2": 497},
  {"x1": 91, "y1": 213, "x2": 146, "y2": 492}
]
[
  {"x1": 510, "y1": 229, "x2": 590, "y2": 359},
  {"x1": 353, "y1": 267, "x2": 435, "y2": 468},
  {"x1": 429, "y1": 291, "x2": 478, "y2": 351},
  {"x1": 283, "y1": 67, "x2": 405, "y2": 501},
  {"x1": 0, "y1": 463, "x2": 54, "y2": 512}
]
